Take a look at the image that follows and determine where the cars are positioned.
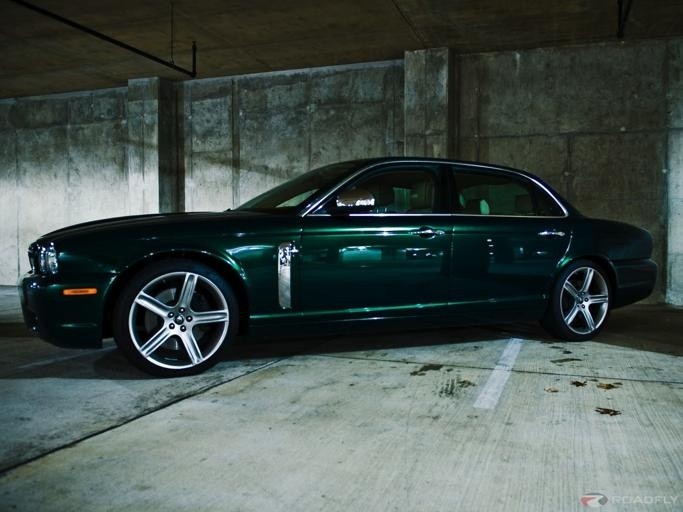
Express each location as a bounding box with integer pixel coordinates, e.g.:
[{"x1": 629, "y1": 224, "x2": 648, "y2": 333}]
[{"x1": 18, "y1": 154, "x2": 659, "y2": 377}]
